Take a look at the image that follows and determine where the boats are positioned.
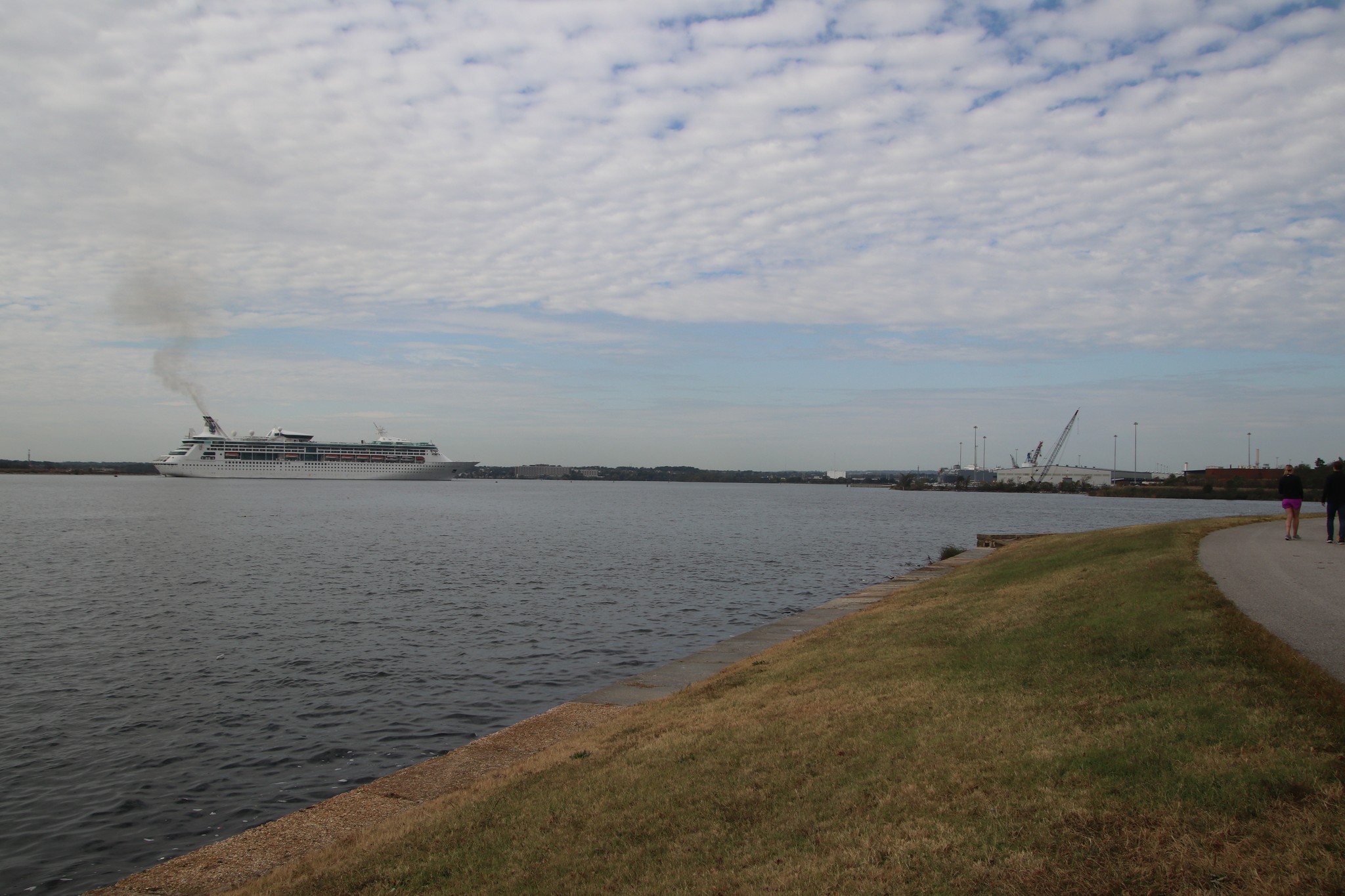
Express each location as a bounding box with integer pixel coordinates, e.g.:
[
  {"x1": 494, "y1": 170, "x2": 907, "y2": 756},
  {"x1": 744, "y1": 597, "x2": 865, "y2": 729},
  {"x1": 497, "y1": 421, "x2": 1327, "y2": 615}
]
[
  {"x1": 225, "y1": 452, "x2": 239, "y2": 457},
  {"x1": 285, "y1": 454, "x2": 298, "y2": 459},
  {"x1": 325, "y1": 455, "x2": 424, "y2": 462}
]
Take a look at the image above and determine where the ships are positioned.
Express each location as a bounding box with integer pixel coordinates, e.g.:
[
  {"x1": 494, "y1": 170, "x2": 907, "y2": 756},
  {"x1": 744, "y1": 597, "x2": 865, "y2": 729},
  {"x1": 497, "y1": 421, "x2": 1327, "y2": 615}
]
[{"x1": 150, "y1": 415, "x2": 481, "y2": 481}]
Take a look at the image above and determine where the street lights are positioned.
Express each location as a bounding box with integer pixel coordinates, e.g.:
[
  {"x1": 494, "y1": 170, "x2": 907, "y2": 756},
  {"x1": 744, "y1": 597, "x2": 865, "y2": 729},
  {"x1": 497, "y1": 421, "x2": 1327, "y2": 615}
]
[
  {"x1": 1275, "y1": 456, "x2": 1279, "y2": 469},
  {"x1": 1288, "y1": 459, "x2": 1292, "y2": 466},
  {"x1": 1077, "y1": 455, "x2": 1081, "y2": 468},
  {"x1": 983, "y1": 436, "x2": 987, "y2": 480},
  {"x1": 959, "y1": 442, "x2": 963, "y2": 478},
  {"x1": 1247, "y1": 432, "x2": 1251, "y2": 468},
  {"x1": 1113, "y1": 435, "x2": 1118, "y2": 486},
  {"x1": 974, "y1": 425, "x2": 978, "y2": 484},
  {"x1": 975, "y1": 445, "x2": 978, "y2": 483},
  {"x1": 1133, "y1": 421, "x2": 1138, "y2": 482},
  {"x1": 1015, "y1": 449, "x2": 1019, "y2": 467},
  {"x1": 1157, "y1": 463, "x2": 1168, "y2": 473}
]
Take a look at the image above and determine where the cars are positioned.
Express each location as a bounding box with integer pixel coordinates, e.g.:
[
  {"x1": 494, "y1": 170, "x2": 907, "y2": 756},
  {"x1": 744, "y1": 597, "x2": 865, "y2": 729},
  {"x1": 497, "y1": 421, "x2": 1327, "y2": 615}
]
[{"x1": 924, "y1": 479, "x2": 981, "y2": 489}]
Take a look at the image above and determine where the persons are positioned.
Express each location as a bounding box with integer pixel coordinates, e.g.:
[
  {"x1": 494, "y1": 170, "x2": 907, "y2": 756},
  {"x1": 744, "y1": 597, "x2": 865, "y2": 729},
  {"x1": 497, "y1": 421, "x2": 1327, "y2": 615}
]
[
  {"x1": 1321, "y1": 461, "x2": 1345, "y2": 544},
  {"x1": 1278, "y1": 465, "x2": 1303, "y2": 540}
]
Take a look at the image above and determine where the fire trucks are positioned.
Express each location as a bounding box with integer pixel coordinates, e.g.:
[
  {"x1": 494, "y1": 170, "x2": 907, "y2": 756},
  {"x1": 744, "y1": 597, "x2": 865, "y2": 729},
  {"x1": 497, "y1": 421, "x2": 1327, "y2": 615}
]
[
  {"x1": 1030, "y1": 440, "x2": 1043, "y2": 465},
  {"x1": 1010, "y1": 454, "x2": 1018, "y2": 468}
]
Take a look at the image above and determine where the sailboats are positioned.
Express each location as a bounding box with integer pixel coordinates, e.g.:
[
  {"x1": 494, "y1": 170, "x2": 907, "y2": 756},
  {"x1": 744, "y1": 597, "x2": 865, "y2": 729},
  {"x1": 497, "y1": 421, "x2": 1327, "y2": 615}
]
[{"x1": 113, "y1": 471, "x2": 118, "y2": 477}]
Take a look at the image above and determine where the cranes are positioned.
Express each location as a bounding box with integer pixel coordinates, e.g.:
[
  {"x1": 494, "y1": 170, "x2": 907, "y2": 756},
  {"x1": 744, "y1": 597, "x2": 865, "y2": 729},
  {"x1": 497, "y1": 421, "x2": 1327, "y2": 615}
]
[{"x1": 1030, "y1": 405, "x2": 1080, "y2": 483}]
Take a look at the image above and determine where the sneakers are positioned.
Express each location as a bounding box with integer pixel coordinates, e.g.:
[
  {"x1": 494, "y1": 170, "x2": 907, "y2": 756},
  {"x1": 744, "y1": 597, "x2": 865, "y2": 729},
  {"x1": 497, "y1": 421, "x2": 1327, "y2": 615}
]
[
  {"x1": 1327, "y1": 538, "x2": 1333, "y2": 542},
  {"x1": 1285, "y1": 535, "x2": 1292, "y2": 540},
  {"x1": 1337, "y1": 539, "x2": 1345, "y2": 544},
  {"x1": 1292, "y1": 534, "x2": 1302, "y2": 540}
]
